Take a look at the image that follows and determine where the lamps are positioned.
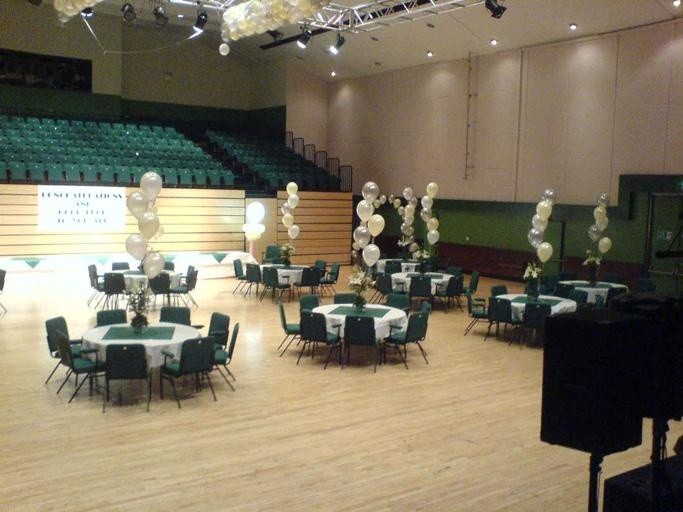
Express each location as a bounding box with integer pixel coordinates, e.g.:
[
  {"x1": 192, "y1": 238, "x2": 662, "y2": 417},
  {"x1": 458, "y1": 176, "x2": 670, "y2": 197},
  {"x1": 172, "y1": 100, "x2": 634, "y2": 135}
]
[
  {"x1": 119, "y1": 2, "x2": 136, "y2": 21},
  {"x1": 267, "y1": 30, "x2": 283, "y2": 41},
  {"x1": 81, "y1": 7, "x2": 93, "y2": 16},
  {"x1": 329, "y1": 32, "x2": 344, "y2": 54},
  {"x1": 296, "y1": 24, "x2": 313, "y2": 48},
  {"x1": 485, "y1": 0, "x2": 507, "y2": 18},
  {"x1": 194, "y1": 7, "x2": 208, "y2": 32},
  {"x1": 153, "y1": 6, "x2": 168, "y2": 25}
]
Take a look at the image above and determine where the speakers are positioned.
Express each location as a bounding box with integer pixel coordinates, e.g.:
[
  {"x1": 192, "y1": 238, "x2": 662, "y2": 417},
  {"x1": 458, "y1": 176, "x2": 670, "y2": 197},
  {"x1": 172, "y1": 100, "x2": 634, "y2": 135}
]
[
  {"x1": 609, "y1": 292, "x2": 683, "y2": 420},
  {"x1": 541, "y1": 306, "x2": 643, "y2": 453}
]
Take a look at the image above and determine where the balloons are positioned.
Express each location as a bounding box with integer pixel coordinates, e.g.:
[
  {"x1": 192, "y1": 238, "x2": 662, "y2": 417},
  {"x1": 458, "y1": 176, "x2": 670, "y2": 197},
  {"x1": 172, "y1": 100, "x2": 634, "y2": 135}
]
[
  {"x1": 124, "y1": 171, "x2": 164, "y2": 281},
  {"x1": 587, "y1": 192, "x2": 612, "y2": 254},
  {"x1": 525, "y1": 188, "x2": 553, "y2": 265},
  {"x1": 53, "y1": 0, "x2": 103, "y2": 26},
  {"x1": 352, "y1": 181, "x2": 440, "y2": 266},
  {"x1": 281, "y1": 182, "x2": 299, "y2": 240},
  {"x1": 218, "y1": 0, "x2": 329, "y2": 57},
  {"x1": 242, "y1": 202, "x2": 266, "y2": 241}
]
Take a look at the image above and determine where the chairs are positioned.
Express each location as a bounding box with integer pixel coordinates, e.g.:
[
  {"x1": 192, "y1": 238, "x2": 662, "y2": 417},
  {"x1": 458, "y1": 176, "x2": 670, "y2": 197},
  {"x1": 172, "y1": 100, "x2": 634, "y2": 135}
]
[
  {"x1": 0, "y1": 269, "x2": 7, "y2": 316},
  {"x1": 232, "y1": 245, "x2": 340, "y2": 303}
]
